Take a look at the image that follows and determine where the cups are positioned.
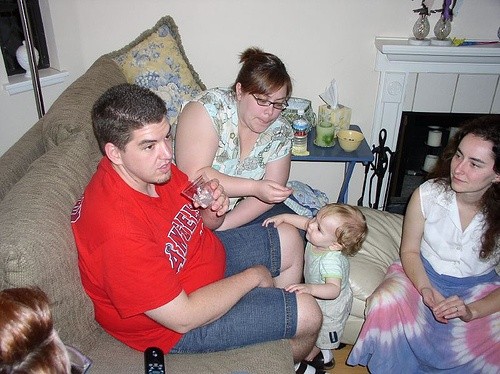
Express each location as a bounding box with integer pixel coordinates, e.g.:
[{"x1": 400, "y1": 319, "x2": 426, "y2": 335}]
[
  {"x1": 182, "y1": 171, "x2": 215, "y2": 209},
  {"x1": 316, "y1": 122, "x2": 335, "y2": 146}
]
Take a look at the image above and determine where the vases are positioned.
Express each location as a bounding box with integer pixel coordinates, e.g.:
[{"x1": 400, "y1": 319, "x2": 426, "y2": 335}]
[
  {"x1": 413, "y1": 0, "x2": 434, "y2": 40},
  {"x1": 434, "y1": 14, "x2": 452, "y2": 40}
]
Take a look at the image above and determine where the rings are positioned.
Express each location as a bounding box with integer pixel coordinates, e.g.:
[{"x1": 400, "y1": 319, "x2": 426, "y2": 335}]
[{"x1": 455, "y1": 306, "x2": 458, "y2": 312}]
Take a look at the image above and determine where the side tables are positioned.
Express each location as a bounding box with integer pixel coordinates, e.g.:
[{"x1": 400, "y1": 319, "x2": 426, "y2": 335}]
[{"x1": 291, "y1": 125, "x2": 373, "y2": 202}]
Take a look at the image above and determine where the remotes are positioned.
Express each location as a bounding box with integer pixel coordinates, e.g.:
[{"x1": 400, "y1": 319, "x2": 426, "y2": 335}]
[{"x1": 144, "y1": 347, "x2": 165, "y2": 374}]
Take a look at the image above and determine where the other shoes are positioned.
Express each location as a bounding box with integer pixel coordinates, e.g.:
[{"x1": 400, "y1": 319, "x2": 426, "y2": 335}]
[{"x1": 317, "y1": 357, "x2": 336, "y2": 369}]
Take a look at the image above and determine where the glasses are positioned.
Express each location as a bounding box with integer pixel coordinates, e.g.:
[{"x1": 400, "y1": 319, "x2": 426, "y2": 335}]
[{"x1": 247, "y1": 87, "x2": 289, "y2": 110}]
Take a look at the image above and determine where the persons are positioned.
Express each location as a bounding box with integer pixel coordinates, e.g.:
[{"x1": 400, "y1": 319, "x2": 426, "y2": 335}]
[
  {"x1": 166, "y1": 48, "x2": 308, "y2": 286},
  {"x1": 0, "y1": 287, "x2": 71, "y2": 374},
  {"x1": 68, "y1": 83, "x2": 324, "y2": 374},
  {"x1": 262, "y1": 204, "x2": 368, "y2": 370},
  {"x1": 346, "y1": 124, "x2": 500, "y2": 374}
]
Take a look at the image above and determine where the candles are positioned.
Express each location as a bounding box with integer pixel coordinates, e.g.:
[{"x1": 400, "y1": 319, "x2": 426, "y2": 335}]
[
  {"x1": 428, "y1": 130, "x2": 442, "y2": 146},
  {"x1": 424, "y1": 154, "x2": 439, "y2": 171}
]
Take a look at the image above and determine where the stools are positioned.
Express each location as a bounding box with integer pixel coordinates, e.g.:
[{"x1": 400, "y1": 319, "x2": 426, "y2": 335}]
[{"x1": 304, "y1": 206, "x2": 404, "y2": 345}]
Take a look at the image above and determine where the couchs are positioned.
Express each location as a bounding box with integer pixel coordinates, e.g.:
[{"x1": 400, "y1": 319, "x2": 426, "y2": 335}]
[{"x1": 0, "y1": 55, "x2": 296, "y2": 374}]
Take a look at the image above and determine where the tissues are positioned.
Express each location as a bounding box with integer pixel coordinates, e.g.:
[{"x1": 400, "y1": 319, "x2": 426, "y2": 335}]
[{"x1": 318, "y1": 79, "x2": 352, "y2": 138}]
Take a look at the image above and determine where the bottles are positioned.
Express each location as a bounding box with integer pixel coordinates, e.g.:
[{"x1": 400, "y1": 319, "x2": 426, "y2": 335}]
[{"x1": 292, "y1": 110, "x2": 308, "y2": 153}]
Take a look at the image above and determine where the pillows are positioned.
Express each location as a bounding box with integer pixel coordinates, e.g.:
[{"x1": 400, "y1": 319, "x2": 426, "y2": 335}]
[{"x1": 110, "y1": 15, "x2": 207, "y2": 125}]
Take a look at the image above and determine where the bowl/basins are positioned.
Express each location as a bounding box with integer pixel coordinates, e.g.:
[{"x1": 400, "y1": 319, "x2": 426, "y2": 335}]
[{"x1": 336, "y1": 129, "x2": 364, "y2": 152}]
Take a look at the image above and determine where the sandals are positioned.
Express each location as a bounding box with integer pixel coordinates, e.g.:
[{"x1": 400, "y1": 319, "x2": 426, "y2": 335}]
[{"x1": 293, "y1": 362, "x2": 327, "y2": 374}]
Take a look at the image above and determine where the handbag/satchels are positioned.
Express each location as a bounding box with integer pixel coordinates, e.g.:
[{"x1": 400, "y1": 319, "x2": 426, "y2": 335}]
[{"x1": 281, "y1": 98, "x2": 313, "y2": 132}]
